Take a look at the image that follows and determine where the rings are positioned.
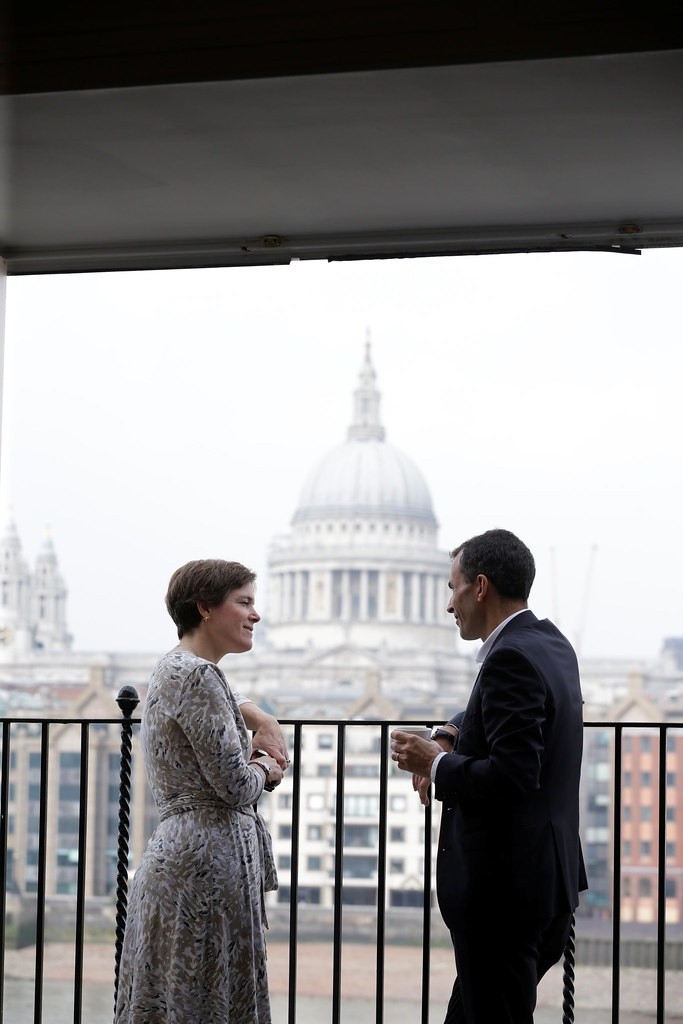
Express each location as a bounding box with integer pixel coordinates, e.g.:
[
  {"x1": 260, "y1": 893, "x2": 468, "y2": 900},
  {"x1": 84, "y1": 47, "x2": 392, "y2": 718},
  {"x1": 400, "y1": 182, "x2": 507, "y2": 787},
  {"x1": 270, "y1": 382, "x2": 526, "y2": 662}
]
[
  {"x1": 396, "y1": 753, "x2": 400, "y2": 761},
  {"x1": 285, "y1": 759, "x2": 291, "y2": 763}
]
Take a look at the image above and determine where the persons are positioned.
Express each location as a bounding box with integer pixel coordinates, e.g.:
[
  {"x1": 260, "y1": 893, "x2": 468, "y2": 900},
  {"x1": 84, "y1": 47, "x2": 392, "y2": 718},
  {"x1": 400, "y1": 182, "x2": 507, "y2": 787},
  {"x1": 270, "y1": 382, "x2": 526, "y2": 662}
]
[
  {"x1": 112, "y1": 559, "x2": 291, "y2": 1023},
  {"x1": 391, "y1": 529, "x2": 588, "y2": 1023}
]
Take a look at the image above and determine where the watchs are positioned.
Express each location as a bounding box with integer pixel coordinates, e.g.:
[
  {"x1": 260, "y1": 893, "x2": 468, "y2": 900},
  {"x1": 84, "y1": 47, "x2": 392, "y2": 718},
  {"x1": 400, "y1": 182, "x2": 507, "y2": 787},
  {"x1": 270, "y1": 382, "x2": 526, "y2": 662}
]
[
  {"x1": 247, "y1": 760, "x2": 271, "y2": 777},
  {"x1": 431, "y1": 728, "x2": 455, "y2": 747}
]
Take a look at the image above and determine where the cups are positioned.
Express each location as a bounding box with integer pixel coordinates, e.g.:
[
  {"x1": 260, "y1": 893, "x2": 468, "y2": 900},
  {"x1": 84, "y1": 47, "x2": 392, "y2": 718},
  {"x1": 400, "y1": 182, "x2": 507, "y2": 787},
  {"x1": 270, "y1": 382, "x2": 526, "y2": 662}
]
[{"x1": 394, "y1": 727, "x2": 432, "y2": 745}]
